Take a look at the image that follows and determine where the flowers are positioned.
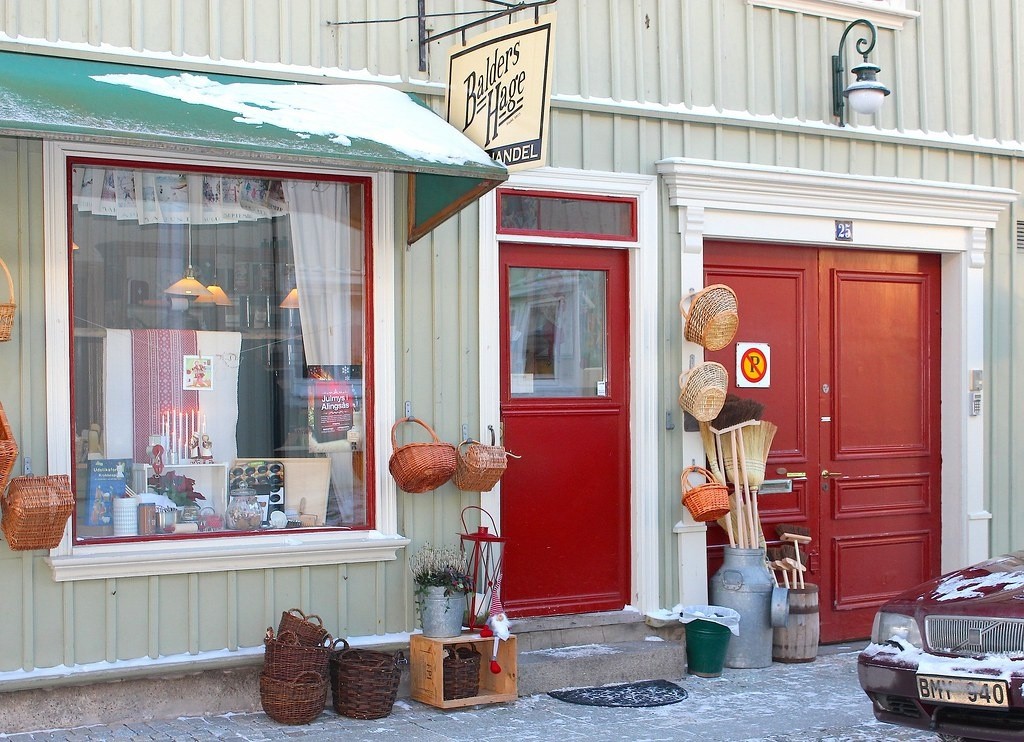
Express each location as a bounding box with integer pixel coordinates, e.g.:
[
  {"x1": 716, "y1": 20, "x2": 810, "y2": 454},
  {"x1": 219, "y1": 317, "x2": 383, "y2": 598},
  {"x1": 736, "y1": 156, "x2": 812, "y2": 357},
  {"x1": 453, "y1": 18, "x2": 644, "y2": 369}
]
[
  {"x1": 408, "y1": 541, "x2": 475, "y2": 629},
  {"x1": 147, "y1": 470, "x2": 206, "y2": 510}
]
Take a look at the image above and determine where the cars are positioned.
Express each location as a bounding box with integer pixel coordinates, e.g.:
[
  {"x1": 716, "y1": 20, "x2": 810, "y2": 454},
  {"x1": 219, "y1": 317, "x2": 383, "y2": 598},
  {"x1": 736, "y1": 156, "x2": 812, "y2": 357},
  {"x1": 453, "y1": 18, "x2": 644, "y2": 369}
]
[{"x1": 857, "y1": 550, "x2": 1024, "y2": 742}]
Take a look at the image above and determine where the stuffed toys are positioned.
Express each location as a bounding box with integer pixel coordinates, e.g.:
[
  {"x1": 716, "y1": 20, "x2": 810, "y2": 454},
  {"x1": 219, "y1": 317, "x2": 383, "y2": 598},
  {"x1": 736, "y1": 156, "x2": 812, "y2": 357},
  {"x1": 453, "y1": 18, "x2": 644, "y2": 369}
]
[{"x1": 480, "y1": 594, "x2": 512, "y2": 674}]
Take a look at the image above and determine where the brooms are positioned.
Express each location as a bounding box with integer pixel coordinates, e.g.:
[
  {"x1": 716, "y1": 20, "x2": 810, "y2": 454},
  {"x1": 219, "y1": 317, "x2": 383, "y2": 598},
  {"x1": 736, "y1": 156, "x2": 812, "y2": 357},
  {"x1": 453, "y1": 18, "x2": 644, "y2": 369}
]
[
  {"x1": 766, "y1": 525, "x2": 812, "y2": 590},
  {"x1": 709, "y1": 398, "x2": 763, "y2": 550},
  {"x1": 698, "y1": 418, "x2": 777, "y2": 549}
]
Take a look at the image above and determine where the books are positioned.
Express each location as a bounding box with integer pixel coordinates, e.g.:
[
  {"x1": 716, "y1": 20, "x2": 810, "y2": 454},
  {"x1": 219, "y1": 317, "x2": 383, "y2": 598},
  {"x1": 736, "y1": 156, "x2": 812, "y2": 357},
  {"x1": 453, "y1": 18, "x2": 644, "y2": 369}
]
[{"x1": 85, "y1": 459, "x2": 133, "y2": 526}]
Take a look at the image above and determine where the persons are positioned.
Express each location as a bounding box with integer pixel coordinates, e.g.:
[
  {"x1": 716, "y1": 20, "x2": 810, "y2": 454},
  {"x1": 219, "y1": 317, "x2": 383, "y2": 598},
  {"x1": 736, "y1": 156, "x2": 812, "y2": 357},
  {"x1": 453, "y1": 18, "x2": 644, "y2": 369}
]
[
  {"x1": 189, "y1": 433, "x2": 201, "y2": 464},
  {"x1": 202, "y1": 433, "x2": 214, "y2": 464}
]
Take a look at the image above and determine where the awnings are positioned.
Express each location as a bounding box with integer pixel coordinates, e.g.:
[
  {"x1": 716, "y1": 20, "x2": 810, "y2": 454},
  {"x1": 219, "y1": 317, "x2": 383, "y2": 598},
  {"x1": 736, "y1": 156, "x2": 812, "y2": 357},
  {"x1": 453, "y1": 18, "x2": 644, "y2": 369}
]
[{"x1": 0, "y1": 51, "x2": 509, "y2": 245}]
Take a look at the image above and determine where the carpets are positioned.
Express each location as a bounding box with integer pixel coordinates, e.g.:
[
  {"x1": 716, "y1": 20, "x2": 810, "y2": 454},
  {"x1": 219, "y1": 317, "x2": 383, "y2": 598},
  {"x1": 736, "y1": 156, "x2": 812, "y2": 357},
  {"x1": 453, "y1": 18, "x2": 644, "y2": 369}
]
[{"x1": 549, "y1": 679, "x2": 688, "y2": 708}]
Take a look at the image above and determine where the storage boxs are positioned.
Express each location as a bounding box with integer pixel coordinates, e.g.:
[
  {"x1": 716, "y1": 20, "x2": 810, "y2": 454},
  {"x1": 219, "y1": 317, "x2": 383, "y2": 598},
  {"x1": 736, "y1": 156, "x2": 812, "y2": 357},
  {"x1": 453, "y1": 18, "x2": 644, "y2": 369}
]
[
  {"x1": 132, "y1": 463, "x2": 227, "y2": 534},
  {"x1": 83, "y1": 459, "x2": 128, "y2": 525},
  {"x1": 410, "y1": 634, "x2": 518, "y2": 709}
]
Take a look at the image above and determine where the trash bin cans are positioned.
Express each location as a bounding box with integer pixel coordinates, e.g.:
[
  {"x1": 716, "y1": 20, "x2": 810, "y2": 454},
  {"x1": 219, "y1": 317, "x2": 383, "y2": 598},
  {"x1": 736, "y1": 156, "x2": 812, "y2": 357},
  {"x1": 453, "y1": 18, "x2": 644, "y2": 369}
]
[{"x1": 682, "y1": 606, "x2": 738, "y2": 678}]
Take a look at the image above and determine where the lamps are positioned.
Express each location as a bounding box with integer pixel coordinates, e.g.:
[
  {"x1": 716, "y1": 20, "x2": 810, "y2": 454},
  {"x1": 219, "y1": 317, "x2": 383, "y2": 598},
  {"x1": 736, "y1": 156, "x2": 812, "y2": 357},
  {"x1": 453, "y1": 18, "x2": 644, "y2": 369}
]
[
  {"x1": 280, "y1": 183, "x2": 299, "y2": 308},
  {"x1": 455, "y1": 506, "x2": 510, "y2": 632},
  {"x1": 832, "y1": 19, "x2": 891, "y2": 128},
  {"x1": 164, "y1": 174, "x2": 233, "y2": 306}
]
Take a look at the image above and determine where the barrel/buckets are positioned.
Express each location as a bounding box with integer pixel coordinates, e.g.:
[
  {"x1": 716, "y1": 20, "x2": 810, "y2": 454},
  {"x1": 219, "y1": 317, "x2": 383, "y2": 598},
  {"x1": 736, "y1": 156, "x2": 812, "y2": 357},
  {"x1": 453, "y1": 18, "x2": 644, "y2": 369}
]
[
  {"x1": 682, "y1": 606, "x2": 739, "y2": 678},
  {"x1": 772, "y1": 582, "x2": 820, "y2": 663}
]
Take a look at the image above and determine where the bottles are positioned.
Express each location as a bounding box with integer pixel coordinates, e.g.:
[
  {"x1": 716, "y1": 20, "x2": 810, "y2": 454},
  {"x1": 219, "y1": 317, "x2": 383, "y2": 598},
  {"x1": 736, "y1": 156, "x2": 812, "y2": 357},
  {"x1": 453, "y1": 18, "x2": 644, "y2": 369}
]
[
  {"x1": 710, "y1": 545, "x2": 789, "y2": 669},
  {"x1": 279, "y1": 236, "x2": 290, "y2": 263},
  {"x1": 270, "y1": 237, "x2": 279, "y2": 262},
  {"x1": 137, "y1": 503, "x2": 156, "y2": 535},
  {"x1": 225, "y1": 481, "x2": 264, "y2": 530},
  {"x1": 260, "y1": 238, "x2": 270, "y2": 262}
]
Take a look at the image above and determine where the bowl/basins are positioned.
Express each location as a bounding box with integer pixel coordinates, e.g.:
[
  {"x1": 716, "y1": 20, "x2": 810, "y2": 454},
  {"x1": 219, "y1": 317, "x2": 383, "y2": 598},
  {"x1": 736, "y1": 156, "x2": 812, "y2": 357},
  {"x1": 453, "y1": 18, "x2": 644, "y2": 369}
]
[
  {"x1": 156, "y1": 511, "x2": 181, "y2": 534},
  {"x1": 175, "y1": 524, "x2": 198, "y2": 534}
]
